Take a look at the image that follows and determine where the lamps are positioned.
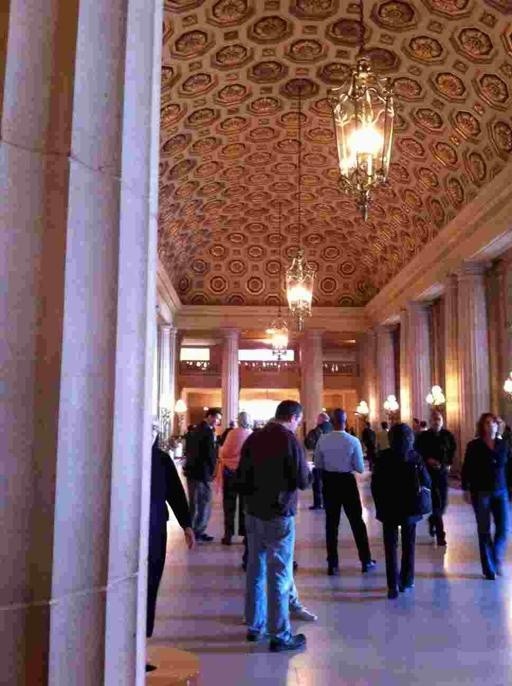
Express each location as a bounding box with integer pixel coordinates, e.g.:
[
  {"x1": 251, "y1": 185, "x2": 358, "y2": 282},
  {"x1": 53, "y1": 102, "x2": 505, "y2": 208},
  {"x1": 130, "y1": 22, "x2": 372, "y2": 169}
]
[
  {"x1": 281, "y1": 77, "x2": 320, "y2": 326},
  {"x1": 268, "y1": 290, "x2": 293, "y2": 355},
  {"x1": 332, "y1": 1, "x2": 397, "y2": 221}
]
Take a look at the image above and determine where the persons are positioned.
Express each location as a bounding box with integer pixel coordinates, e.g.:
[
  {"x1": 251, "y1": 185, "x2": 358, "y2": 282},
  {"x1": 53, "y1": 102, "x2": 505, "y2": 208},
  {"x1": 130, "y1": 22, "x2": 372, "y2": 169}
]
[
  {"x1": 305, "y1": 413, "x2": 334, "y2": 509},
  {"x1": 314, "y1": 409, "x2": 376, "y2": 575},
  {"x1": 460, "y1": 413, "x2": 512, "y2": 579},
  {"x1": 183, "y1": 401, "x2": 317, "y2": 653},
  {"x1": 371, "y1": 423, "x2": 432, "y2": 599},
  {"x1": 145, "y1": 417, "x2": 196, "y2": 672},
  {"x1": 345, "y1": 418, "x2": 429, "y2": 471},
  {"x1": 496, "y1": 415, "x2": 512, "y2": 444},
  {"x1": 413, "y1": 412, "x2": 456, "y2": 545}
]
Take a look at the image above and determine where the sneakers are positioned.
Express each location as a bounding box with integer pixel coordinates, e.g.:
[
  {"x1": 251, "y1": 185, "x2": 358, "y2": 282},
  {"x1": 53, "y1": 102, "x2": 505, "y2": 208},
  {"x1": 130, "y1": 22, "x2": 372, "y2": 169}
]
[
  {"x1": 245, "y1": 604, "x2": 317, "y2": 652},
  {"x1": 195, "y1": 533, "x2": 213, "y2": 542}
]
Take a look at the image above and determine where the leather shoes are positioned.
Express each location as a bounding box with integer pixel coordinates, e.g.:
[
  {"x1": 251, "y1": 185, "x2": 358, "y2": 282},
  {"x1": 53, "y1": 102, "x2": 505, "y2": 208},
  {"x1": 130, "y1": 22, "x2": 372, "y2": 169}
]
[
  {"x1": 361, "y1": 560, "x2": 377, "y2": 571},
  {"x1": 328, "y1": 567, "x2": 339, "y2": 575}
]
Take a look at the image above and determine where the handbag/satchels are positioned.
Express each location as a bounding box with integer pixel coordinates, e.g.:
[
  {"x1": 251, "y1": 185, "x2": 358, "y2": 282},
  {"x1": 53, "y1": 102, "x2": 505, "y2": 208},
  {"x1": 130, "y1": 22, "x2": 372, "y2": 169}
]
[{"x1": 407, "y1": 463, "x2": 433, "y2": 515}]
